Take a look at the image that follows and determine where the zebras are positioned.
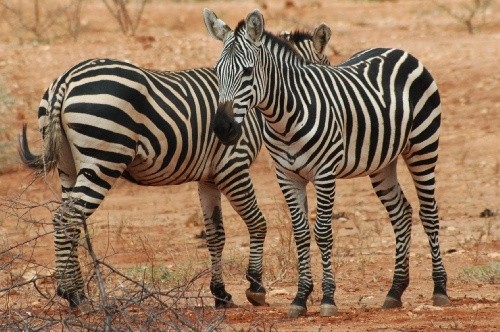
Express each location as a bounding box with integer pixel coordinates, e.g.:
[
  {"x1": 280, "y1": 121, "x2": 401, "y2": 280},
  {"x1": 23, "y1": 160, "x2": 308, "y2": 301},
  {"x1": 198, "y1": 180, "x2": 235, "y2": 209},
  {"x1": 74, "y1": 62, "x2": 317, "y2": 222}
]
[
  {"x1": 202, "y1": 6, "x2": 456, "y2": 319},
  {"x1": 15, "y1": 22, "x2": 333, "y2": 317}
]
